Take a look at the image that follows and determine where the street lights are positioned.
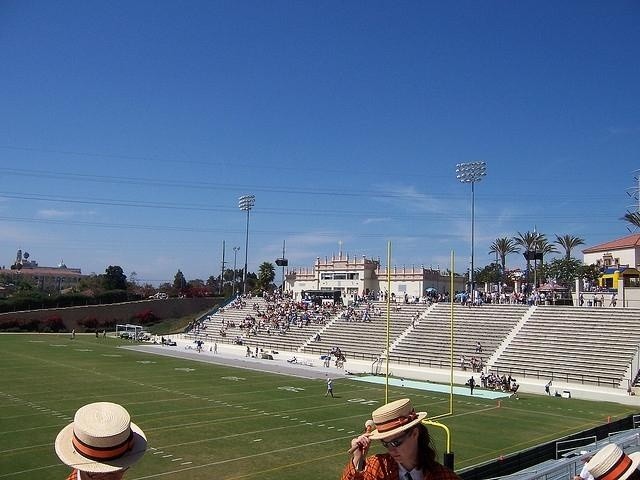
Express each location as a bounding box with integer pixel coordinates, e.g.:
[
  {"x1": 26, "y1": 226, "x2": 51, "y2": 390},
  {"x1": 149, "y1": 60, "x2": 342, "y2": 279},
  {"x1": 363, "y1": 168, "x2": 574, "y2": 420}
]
[
  {"x1": 238, "y1": 194, "x2": 255, "y2": 296},
  {"x1": 455, "y1": 159, "x2": 487, "y2": 303},
  {"x1": 232, "y1": 247, "x2": 241, "y2": 295}
]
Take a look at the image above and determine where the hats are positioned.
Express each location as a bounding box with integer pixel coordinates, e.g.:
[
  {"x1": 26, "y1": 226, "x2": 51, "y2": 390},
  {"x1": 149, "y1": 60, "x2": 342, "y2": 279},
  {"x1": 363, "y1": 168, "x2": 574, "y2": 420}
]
[{"x1": 367, "y1": 399, "x2": 427, "y2": 440}]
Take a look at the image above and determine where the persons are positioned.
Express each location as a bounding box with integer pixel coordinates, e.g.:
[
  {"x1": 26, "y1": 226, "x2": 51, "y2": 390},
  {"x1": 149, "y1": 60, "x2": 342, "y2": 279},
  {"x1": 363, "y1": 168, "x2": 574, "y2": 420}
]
[
  {"x1": 573, "y1": 452, "x2": 595, "y2": 480},
  {"x1": 459, "y1": 354, "x2": 519, "y2": 399},
  {"x1": 475, "y1": 342, "x2": 483, "y2": 353},
  {"x1": 72, "y1": 327, "x2": 76, "y2": 340},
  {"x1": 103, "y1": 329, "x2": 106, "y2": 337},
  {"x1": 145, "y1": 286, "x2": 422, "y2": 368},
  {"x1": 422, "y1": 282, "x2": 619, "y2": 308},
  {"x1": 325, "y1": 377, "x2": 334, "y2": 397},
  {"x1": 340, "y1": 397, "x2": 460, "y2": 480},
  {"x1": 95, "y1": 330, "x2": 99, "y2": 339},
  {"x1": 544, "y1": 380, "x2": 552, "y2": 396}
]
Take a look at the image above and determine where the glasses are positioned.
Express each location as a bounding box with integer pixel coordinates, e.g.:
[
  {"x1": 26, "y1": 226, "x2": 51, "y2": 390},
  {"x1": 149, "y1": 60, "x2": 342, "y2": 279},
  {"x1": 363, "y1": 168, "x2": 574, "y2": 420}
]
[
  {"x1": 85, "y1": 467, "x2": 129, "y2": 479},
  {"x1": 380, "y1": 431, "x2": 411, "y2": 448}
]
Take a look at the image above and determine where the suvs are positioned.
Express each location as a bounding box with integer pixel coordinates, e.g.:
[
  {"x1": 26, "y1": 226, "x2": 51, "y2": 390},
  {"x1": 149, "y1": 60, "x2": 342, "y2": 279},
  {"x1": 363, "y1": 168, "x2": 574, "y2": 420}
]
[{"x1": 148, "y1": 293, "x2": 167, "y2": 300}]
